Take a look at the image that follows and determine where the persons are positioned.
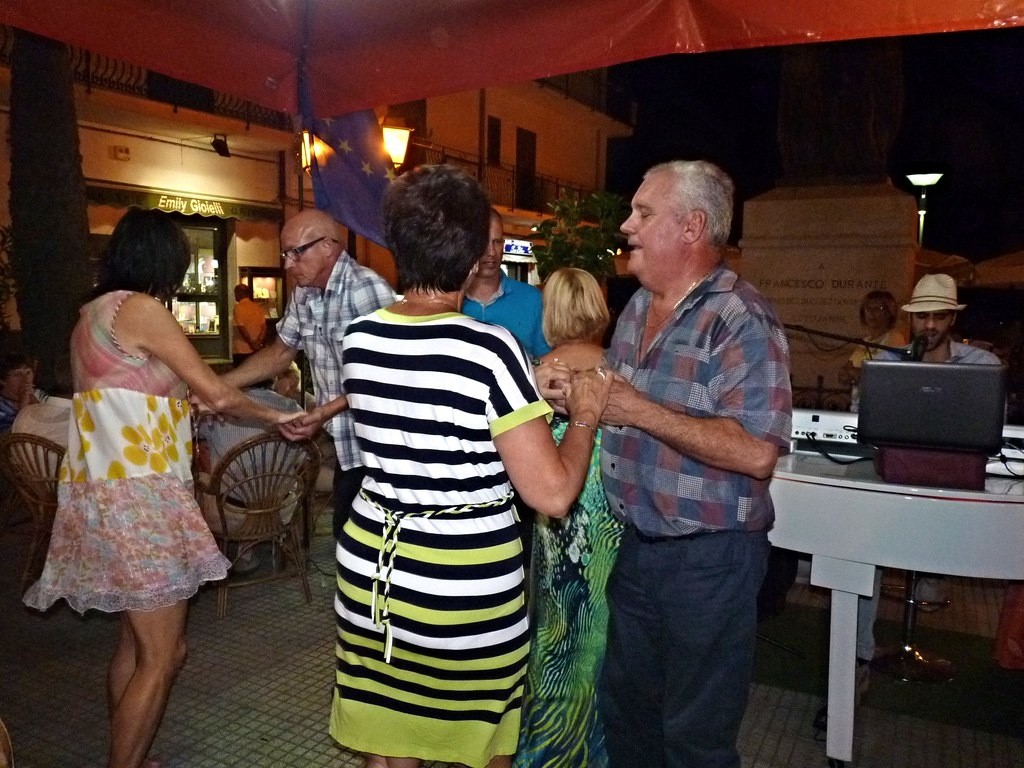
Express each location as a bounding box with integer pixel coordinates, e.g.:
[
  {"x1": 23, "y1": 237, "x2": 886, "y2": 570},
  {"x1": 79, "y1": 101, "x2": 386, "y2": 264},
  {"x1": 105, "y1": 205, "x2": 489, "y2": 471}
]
[
  {"x1": 840, "y1": 271, "x2": 1001, "y2": 702},
  {"x1": 21, "y1": 203, "x2": 306, "y2": 768},
  {"x1": 0, "y1": 337, "x2": 318, "y2": 574},
  {"x1": 234, "y1": 284, "x2": 268, "y2": 389},
  {"x1": 326, "y1": 158, "x2": 614, "y2": 768},
  {"x1": 549, "y1": 156, "x2": 794, "y2": 768},
  {"x1": 512, "y1": 266, "x2": 624, "y2": 768},
  {"x1": 190, "y1": 207, "x2": 400, "y2": 534},
  {"x1": 459, "y1": 207, "x2": 552, "y2": 570}
]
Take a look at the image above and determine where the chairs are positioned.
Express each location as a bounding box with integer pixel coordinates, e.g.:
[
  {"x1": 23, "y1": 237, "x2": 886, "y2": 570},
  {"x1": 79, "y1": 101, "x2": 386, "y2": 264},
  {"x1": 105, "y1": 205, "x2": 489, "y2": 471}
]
[
  {"x1": 0, "y1": 432, "x2": 65, "y2": 597},
  {"x1": 193, "y1": 434, "x2": 323, "y2": 619}
]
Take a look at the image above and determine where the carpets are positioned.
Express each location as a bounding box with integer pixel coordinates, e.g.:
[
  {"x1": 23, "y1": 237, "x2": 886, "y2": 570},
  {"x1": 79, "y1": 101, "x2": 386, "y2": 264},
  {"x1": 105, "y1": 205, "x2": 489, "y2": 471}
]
[{"x1": 749, "y1": 604, "x2": 1024, "y2": 739}]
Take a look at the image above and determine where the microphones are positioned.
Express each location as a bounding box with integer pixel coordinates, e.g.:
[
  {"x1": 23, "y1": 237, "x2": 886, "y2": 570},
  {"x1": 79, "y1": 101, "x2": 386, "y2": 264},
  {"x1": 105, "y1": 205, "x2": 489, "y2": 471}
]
[{"x1": 912, "y1": 335, "x2": 929, "y2": 362}]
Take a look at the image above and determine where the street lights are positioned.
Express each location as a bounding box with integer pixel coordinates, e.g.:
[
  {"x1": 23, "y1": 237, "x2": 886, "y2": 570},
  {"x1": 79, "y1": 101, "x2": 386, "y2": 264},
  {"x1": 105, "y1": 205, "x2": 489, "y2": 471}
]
[
  {"x1": 906, "y1": 173, "x2": 943, "y2": 248},
  {"x1": 296, "y1": 113, "x2": 416, "y2": 263}
]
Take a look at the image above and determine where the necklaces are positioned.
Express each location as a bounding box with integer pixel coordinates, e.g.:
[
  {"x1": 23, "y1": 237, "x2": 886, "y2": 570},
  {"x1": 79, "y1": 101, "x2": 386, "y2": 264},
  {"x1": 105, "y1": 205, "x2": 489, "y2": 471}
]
[{"x1": 645, "y1": 264, "x2": 718, "y2": 329}]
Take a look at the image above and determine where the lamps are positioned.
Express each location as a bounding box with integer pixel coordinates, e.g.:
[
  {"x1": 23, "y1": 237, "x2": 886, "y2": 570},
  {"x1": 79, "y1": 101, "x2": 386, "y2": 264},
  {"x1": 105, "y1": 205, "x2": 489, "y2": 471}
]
[{"x1": 181, "y1": 133, "x2": 231, "y2": 157}]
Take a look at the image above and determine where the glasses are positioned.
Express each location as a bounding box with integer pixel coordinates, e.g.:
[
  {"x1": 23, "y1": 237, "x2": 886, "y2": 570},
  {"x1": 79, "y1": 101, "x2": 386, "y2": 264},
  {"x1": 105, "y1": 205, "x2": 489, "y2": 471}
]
[
  {"x1": 280, "y1": 236, "x2": 340, "y2": 261},
  {"x1": 864, "y1": 304, "x2": 888, "y2": 312}
]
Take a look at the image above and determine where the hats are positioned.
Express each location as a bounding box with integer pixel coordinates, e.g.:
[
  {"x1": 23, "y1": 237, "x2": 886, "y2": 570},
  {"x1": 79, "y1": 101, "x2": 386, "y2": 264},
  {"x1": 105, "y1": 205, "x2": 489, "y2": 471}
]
[{"x1": 902, "y1": 272, "x2": 966, "y2": 311}]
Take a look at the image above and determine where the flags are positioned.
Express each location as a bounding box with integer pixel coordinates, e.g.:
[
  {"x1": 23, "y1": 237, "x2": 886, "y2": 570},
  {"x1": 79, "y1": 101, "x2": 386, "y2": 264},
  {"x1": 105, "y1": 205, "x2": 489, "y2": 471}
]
[{"x1": 297, "y1": 49, "x2": 394, "y2": 251}]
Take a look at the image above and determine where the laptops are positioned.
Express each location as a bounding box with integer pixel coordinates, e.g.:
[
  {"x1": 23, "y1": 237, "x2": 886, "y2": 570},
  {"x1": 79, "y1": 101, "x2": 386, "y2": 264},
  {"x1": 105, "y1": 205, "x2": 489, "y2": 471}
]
[{"x1": 858, "y1": 359, "x2": 1008, "y2": 455}]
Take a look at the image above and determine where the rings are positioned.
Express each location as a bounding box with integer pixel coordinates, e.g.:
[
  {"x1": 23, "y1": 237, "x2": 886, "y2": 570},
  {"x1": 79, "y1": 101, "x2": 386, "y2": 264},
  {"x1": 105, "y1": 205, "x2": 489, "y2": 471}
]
[
  {"x1": 597, "y1": 368, "x2": 606, "y2": 378},
  {"x1": 554, "y1": 357, "x2": 560, "y2": 362}
]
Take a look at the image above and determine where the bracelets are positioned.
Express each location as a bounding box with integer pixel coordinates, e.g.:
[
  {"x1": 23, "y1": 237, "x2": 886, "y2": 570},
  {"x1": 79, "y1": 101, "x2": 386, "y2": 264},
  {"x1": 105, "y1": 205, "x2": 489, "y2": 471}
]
[{"x1": 566, "y1": 420, "x2": 597, "y2": 437}]
